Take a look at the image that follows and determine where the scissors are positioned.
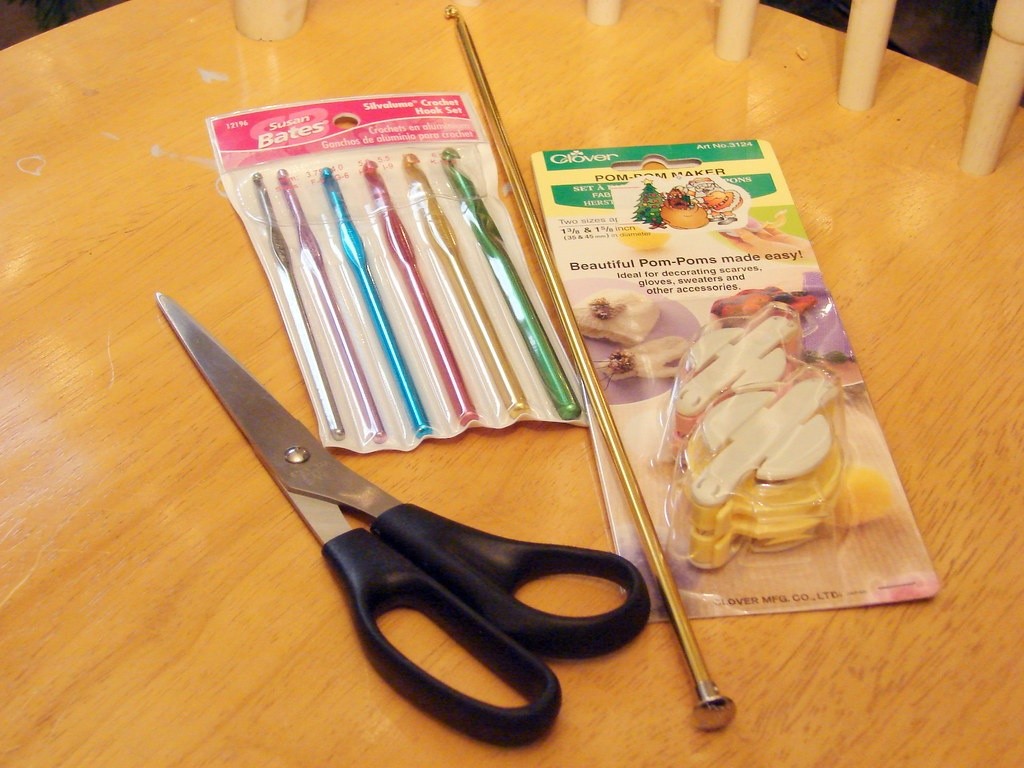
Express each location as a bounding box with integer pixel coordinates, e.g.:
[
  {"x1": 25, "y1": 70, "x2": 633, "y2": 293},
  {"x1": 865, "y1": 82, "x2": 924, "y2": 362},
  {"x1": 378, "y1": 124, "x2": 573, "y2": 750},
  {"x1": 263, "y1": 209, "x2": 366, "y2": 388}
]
[{"x1": 154, "y1": 287, "x2": 654, "y2": 748}]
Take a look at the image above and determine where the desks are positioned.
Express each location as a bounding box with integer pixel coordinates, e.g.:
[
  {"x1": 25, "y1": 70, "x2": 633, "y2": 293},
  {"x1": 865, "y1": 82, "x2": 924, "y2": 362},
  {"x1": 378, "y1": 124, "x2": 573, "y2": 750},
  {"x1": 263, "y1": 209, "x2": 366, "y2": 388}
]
[{"x1": 0, "y1": 0, "x2": 1024, "y2": 768}]
